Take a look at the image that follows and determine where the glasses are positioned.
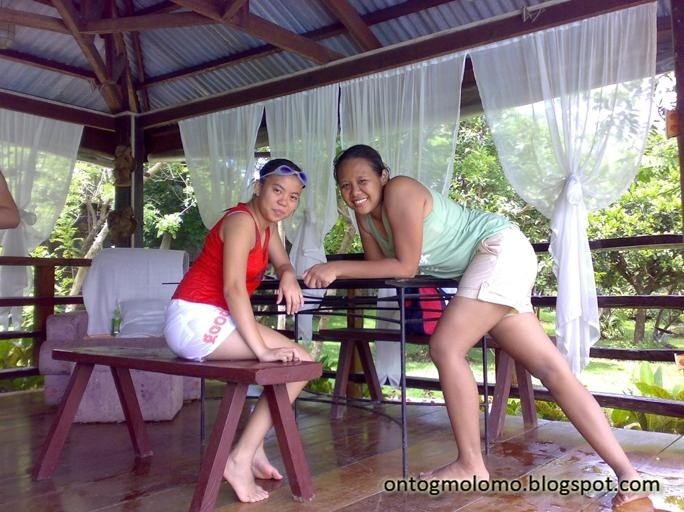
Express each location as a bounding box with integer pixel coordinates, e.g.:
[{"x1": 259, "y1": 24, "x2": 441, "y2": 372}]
[{"x1": 257, "y1": 166, "x2": 308, "y2": 187}]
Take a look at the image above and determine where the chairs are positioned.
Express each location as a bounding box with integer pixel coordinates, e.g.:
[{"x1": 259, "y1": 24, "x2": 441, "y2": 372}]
[{"x1": 39, "y1": 247, "x2": 202, "y2": 424}]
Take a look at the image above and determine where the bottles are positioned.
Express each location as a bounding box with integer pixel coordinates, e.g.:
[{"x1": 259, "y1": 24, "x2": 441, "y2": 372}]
[{"x1": 111, "y1": 308, "x2": 122, "y2": 336}]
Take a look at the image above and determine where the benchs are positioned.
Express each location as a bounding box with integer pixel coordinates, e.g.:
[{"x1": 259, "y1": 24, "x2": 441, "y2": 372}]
[
  {"x1": 32, "y1": 346, "x2": 322, "y2": 511},
  {"x1": 320, "y1": 328, "x2": 555, "y2": 442}
]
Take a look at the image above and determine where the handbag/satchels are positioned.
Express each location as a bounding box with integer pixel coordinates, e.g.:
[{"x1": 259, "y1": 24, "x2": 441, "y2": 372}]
[{"x1": 398, "y1": 282, "x2": 448, "y2": 336}]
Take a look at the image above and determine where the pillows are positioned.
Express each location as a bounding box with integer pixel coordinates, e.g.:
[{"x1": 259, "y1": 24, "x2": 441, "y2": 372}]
[{"x1": 116, "y1": 299, "x2": 166, "y2": 339}]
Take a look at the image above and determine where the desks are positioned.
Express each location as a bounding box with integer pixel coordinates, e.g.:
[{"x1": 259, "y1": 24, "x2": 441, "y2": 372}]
[{"x1": 162, "y1": 275, "x2": 488, "y2": 478}]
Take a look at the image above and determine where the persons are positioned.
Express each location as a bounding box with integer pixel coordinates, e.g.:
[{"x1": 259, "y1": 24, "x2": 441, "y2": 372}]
[
  {"x1": 0, "y1": 170, "x2": 23, "y2": 228},
  {"x1": 303, "y1": 145, "x2": 661, "y2": 509},
  {"x1": 162, "y1": 159, "x2": 314, "y2": 502},
  {"x1": 64, "y1": 206, "x2": 139, "y2": 312}
]
